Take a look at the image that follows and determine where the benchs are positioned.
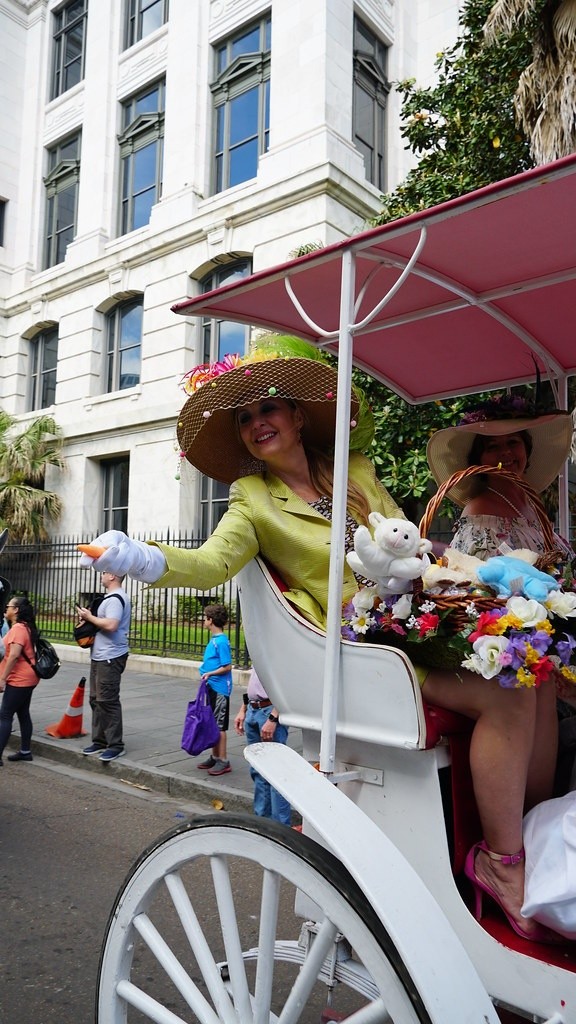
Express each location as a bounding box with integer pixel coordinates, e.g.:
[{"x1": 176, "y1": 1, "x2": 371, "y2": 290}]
[{"x1": 236, "y1": 553, "x2": 474, "y2": 750}]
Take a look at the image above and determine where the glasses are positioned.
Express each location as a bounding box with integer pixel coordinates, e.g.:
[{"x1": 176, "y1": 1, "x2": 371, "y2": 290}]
[
  {"x1": 5, "y1": 604, "x2": 20, "y2": 610},
  {"x1": 103, "y1": 571, "x2": 106, "y2": 576}
]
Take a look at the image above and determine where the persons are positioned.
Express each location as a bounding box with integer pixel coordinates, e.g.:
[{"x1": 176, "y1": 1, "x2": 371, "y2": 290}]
[
  {"x1": 75, "y1": 340, "x2": 561, "y2": 941},
  {"x1": 430, "y1": 397, "x2": 574, "y2": 796},
  {"x1": 197, "y1": 605, "x2": 233, "y2": 775},
  {"x1": 74, "y1": 572, "x2": 131, "y2": 760},
  {"x1": 0, "y1": 597, "x2": 39, "y2": 767},
  {"x1": 234, "y1": 667, "x2": 289, "y2": 828}
]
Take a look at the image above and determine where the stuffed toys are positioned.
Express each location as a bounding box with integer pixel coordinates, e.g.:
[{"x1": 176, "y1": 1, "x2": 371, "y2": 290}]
[
  {"x1": 344, "y1": 511, "x2": 433, "y2": 592},
  {"x1": 422, "y1": 546, "x2": 559, "y2": 604}
]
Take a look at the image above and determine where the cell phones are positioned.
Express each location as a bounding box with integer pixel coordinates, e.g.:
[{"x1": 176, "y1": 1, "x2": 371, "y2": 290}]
[{"x1": 75, "y1": 602, "x2": 81, "y2": 609}]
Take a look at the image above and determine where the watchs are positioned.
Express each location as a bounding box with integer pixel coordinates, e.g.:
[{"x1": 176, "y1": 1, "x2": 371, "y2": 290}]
[{"x1": 268, "y1": 714, "x2": 278, "y2": 722}]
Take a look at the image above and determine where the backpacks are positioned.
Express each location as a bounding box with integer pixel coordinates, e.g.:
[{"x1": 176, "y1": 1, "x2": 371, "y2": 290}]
[
  {"x1": 15, "y1": 622, "x2": 60, "y2": 679},
  {"x1": 74, "y1": 593, "x2": 125, "y2": 649}
]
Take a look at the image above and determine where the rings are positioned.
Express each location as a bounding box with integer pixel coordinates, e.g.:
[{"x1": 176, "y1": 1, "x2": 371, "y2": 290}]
[{"x1": 268, "y1": 734, "x2": 270, "y2": 736}]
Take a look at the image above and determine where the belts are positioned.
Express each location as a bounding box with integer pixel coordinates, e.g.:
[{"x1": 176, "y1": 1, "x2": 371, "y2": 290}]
[{"x1": 249, "y1": 701, "x2": 272, "y2": 710}]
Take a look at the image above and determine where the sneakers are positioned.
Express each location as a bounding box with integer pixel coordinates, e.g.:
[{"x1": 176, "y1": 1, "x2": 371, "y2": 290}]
[
  {"x1": 83, "y1": 743, "x2": 108, "y2": 755},
  {"x1": 196, "y1": 755, "x2": 216, "y2": 769},
  {"x1": 0, "y1": 760, "x2": 3, "y2": 766},
  {"x1": 207, "y1": 760, "x2": 231, "y2": 775},
  {"x1": 8, "y1": 752, "x2": 33, "y2": 761},
  {"x1": 99, "y1": 747, "x2": 126, "y2": 761}
]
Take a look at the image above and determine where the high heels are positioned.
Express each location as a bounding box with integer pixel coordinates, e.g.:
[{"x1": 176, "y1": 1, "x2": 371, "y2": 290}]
[{"x1": 464, "y1": 840, "x2": 568, "y2": 946}]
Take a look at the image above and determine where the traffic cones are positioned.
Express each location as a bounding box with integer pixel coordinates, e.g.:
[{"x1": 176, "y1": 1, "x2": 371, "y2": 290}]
[{"x1": 44, "y1": 677, "x2": 88, "y2": 739}]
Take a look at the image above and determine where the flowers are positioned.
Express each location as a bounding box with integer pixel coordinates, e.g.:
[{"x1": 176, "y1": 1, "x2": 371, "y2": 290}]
[
  {"x1": 178, "y1": 349, "x2": 279, "y2": 398},
  {"x1": 333, "y1": 555, "x2": 576, "y2": 690},
  {"x1": 455, "y1": 394, "x2": 537, "y2": 425}
]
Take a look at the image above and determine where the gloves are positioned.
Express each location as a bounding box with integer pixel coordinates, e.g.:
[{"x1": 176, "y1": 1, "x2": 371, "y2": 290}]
[{"x1": 78, "y1": 529, "x2": 149, "y2": 575}]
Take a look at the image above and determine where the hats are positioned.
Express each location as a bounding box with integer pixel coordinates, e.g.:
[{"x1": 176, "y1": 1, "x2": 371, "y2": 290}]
[
  {"x1": 426, "y1": 353, "x2": 573, "y2": 509},
  {"x1": 172, "y1": 335, "x2": 375, "y2": 484}
]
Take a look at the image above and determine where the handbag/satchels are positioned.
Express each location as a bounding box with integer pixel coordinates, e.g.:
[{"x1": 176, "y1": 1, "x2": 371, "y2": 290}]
[
  {"x1": 412, "y1": 463, "x2": 562, "y2": 639},
  {"x1": 181, "y1": 680, "x2": 220, "y2": 756}
]
